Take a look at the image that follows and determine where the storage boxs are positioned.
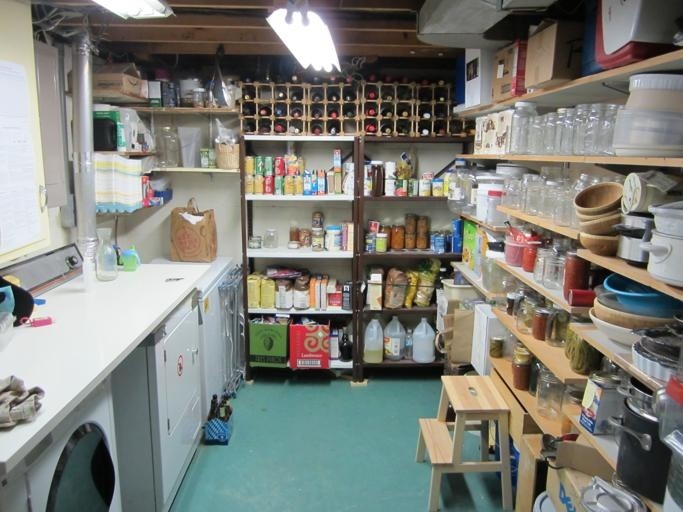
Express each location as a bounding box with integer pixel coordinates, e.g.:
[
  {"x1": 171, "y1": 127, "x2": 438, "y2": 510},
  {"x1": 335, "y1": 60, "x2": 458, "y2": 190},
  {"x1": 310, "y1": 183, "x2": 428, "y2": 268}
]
[
  {"x1": 65, "y1": 62, "x2": 141, "y2": 99},
  {"x1": 544, "y1": 459, "x2": 612, "y2": 512},
  {"x1": 462, "y1": 17, "x2": 583, "y2": 110}
]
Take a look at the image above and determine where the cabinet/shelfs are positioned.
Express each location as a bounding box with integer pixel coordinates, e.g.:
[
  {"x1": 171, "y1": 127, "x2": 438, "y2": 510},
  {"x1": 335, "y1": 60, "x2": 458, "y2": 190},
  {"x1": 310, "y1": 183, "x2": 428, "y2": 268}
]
[
  {"x1": 236, "y1": 135, "x2": 476, "y2": 385},
  {"x1": 449, "y1": 50, "x2": 682, "y2": 512}
]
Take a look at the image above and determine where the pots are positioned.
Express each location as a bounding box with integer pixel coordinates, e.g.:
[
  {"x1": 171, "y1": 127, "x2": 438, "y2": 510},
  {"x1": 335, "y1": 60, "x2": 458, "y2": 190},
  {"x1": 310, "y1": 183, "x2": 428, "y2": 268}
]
[{"x1": 639, "y1": 197, "x2": 682, "y2": 289}]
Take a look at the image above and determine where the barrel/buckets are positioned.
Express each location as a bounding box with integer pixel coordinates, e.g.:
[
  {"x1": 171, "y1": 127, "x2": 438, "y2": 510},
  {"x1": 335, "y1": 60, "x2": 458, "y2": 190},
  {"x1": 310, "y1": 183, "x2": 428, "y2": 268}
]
[
  {"x1": 383, "y1": 315, "x2": 405, "y2": 362},
  {"x1": 412, "y1": 315, "x2": 436, "y2": 364},
  {"x1": 363, "y1": 318, "x2": 384, "y2": 364}
]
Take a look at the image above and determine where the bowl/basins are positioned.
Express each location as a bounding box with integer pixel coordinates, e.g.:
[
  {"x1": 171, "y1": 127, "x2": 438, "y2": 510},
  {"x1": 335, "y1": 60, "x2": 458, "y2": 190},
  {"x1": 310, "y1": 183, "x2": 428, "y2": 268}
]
[
  {"x1": 588, "y1": 271, "x2": 680, "y2": 346},
  {"x1": 573, "y1": 181, "x2": 623, "y2": 258}
]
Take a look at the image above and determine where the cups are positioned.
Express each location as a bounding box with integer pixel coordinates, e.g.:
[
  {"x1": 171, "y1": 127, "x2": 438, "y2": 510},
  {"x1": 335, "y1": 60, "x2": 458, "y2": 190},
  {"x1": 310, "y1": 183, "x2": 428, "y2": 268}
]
[{"x1": 534, "y1": 238, "x2": 574, "y2": 289}]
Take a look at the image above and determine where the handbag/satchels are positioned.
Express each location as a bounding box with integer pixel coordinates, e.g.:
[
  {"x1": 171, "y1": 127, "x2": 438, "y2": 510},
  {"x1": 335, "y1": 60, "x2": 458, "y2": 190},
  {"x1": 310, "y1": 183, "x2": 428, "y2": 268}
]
[
  {"x1": 435, "y1": 310, "x2": 474, "y2": 363},
  {"x1": 171, "y1": 198, "x2": 217, "y2": 263}
]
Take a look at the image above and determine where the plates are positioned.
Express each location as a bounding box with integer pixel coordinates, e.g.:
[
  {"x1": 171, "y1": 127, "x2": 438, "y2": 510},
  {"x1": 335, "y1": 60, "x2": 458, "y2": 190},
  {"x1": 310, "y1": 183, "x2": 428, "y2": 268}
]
[{"x1": 639, "y1": 314, "x2": 683, "y2": 365}]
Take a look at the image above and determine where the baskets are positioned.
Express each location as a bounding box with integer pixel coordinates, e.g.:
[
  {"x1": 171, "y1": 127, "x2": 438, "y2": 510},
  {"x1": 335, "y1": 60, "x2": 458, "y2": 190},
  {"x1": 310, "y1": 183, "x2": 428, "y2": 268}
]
[{"x1": 216, "y1": 143, "x2": 240, "y2": 169}]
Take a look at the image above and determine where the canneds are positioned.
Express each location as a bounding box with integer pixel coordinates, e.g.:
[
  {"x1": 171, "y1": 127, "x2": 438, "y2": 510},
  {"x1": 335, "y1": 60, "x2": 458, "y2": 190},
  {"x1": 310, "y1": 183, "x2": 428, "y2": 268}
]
[
  {"x1": 379, "y1": 224, "x2": 391, "y2": 249},
  {"x1": 533, "y1": 248, "x2": 555, "y2": 284},
  {"x1": 263, "y1": 228, "x2": 279, "y2": 249},
  {"x1": 555, "y1": 308, "x2": 603, "y2": 375},
  {"x1": 416, "y1": 215, "x2": 428, "y2": 232},
  {"x1": 562, "y1": 249, "x2": 590, "y2": 301},
  {"x1": 404, "y1": 213, "x2": 416, "y2": 233},
  {"x1": 391, "y1": 222, "x2": 405, "y2": 249},
  {"x1": 376, "y1": 232, "x2": 387, "y2": 252},
  {"x1": 489, "y1": 336, "x2": 503, "y2": 357},
  {"x1": 533, "y1": 307, "x2": 553, "y2": 341},
  {"x1": 417, "y1": 232, "x2": 427, "y2": 249}
]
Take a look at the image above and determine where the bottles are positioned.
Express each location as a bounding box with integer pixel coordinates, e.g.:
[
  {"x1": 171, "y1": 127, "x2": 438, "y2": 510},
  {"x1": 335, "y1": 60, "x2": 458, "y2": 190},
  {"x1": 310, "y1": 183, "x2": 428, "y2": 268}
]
[
  {"x1": 489, "y1": 322, "x2": 563, "y2": 421},
  {"x1": 503, "y1": 169, "x2": 622, "y2": 230},
  {"x1": 521, "y1": 240, "x2": 541, "y2": 270},
  {"x1": 480, "y1": 260, "x2": 601, "y2": 377},
  {"x1": 81, "y1": 228, "x2": 119, "y2": 284},
  {"x1": 190, "y1": 87, "x2": 207, "y2": 108},
  {"x1": 403, "y1": 329, "x2": 413, "y2": 359},
  {"x1": 509, "y1": 99, "x2": 615, "y2": 155},
  {"x1": 339, "y1": 325, "x2": 354, "y2": 360},
  {"x1": 239, "y1": 74, "x2": 447, "y2": 138},
  {"x1": 263, "y1": 209, "x2": 341, "y2": 253},
  {"x1": 375, "y1": 212, "x2": 429, "y2": 252},
  {"x1": 369, "y1": 160, "x2": 385, "y2": 196},
  {"x1": 206, "y1": 392, "x2": 232, "y2": 422},
  {"x1": 563, "y1": 252, "x2": 595, "y2": 305},
  {"x1": 152, "y1": 125, "x2": 180, "y2": 168}
]
